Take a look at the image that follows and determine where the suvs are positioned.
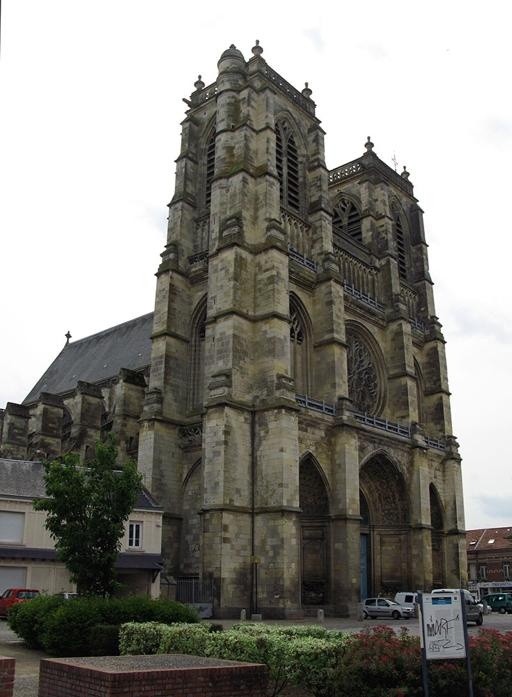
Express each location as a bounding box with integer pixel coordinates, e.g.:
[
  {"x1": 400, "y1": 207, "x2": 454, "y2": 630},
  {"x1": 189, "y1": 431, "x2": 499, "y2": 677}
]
[{"x1": 361, "y1": 598, "x2": 417, "y2": 620}]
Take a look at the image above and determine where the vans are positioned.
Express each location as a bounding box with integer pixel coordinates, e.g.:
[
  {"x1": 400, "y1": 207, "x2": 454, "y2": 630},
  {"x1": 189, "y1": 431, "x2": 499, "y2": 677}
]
[
  {"x1": 393, "y1": 592, "x2": 418, "y2": 617},
  {"x1": 431, "y1": 588, "x2": 485, "y2": 627},
  {"x1": 478, "y1": 592, "x2": 512, "y2": 614}
]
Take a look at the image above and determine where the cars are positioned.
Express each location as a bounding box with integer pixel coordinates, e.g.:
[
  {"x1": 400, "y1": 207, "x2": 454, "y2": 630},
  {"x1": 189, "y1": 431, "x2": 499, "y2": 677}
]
[
  {"x1": 51, "y1": 591, "x2": 83, "y2": 600},
  {"x1": 0, "y1": 587, "x2": 43, "y2": 620}
]
